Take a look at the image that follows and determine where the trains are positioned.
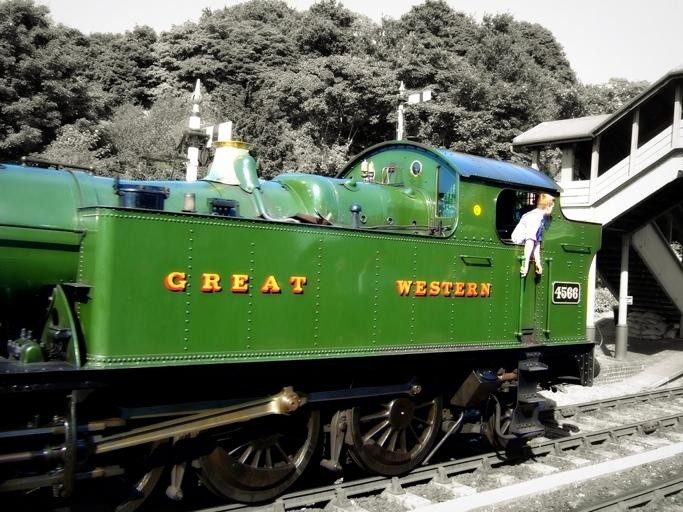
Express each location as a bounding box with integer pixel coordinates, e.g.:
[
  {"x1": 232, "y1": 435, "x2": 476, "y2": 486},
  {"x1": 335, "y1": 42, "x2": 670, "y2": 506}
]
[{"x1": 0, "y1": 76, "x2": 608, "y2": 501}]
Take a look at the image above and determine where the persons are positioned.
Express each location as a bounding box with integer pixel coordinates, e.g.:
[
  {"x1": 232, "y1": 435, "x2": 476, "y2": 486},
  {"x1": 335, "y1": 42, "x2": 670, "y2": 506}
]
[{"x1": 510, "y1": 192, "x2": 555, "y2": 278}]
